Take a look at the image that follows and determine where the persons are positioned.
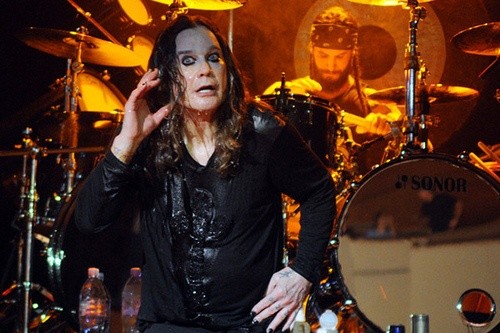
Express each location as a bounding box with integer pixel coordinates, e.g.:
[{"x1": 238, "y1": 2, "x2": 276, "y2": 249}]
[
  {"x1": 359, "y1": 187, "x2": 463, "y2": 276},
  {"x1": 49, "y1": 16, "x2": 337, "y2": 333},
  {"x1": 260, "y1": 7, "x2": 403, "y2": 136}
]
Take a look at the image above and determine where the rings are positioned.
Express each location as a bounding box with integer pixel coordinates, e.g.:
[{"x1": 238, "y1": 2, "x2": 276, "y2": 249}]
[{"x1": 142, "y1": 81, "x2": 148, "y2": 86}]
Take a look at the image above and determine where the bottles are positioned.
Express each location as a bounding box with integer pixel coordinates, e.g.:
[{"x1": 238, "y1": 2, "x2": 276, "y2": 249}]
[
  {"x1": 317, "y1": 309, "x2": 339, "y2": 333},
  {"x1": 121, "y1": 267, "x2": 143, "y2": 333},
  {"x1": 79, "y1": 268, "x2": 107, "y2": 333},
  {"x1": 410, "y1": 313, "x2": 429, "y2": 333},
  {"x1": 97, "y1": 273, "x2": 111, "y2": 333},
  {"x1": 387, "y1": 324, "x2": 406, "y2": 333}
]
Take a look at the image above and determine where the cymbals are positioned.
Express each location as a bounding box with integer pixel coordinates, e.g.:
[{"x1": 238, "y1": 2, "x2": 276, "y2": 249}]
[
  {"x1": 0, "y1": 143, "x2": 106, "y2": 157},
  {"x1": 19, "y1": 27, "x2": 140, "y2": 67},
  {"x1": 369, "y1": 84, "x2": 478, "y2": 107},
  {"x1": 154, "y1": 0, "x2": 245, "y2": 10},
  {"x1": 451, "y1": 21, "x2": 500, "y2": 55},
  {"x1": 71, "y1": 3, "x2": 126, "y2": 58}
]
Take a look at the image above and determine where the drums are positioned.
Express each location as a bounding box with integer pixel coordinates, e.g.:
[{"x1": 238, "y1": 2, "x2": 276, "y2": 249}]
[
  {"x1": 330, "y1": 152, "x2": 500, "y2": 333},
  {"x1": 282, "y1": 167, "x2": 351, "y2": 242},
  {"x1": 94, "y1": 0, "x2": 153, "y2": 43},
  {"x1": 39, "y1": 69, "x2": 135, "y2": 163},
  {"x1": 122, "y1": 32, "x2": 154, "y2": 75},
  {"x1": 254, "y1": 92, "x2": 344, "y2": 175}
]
